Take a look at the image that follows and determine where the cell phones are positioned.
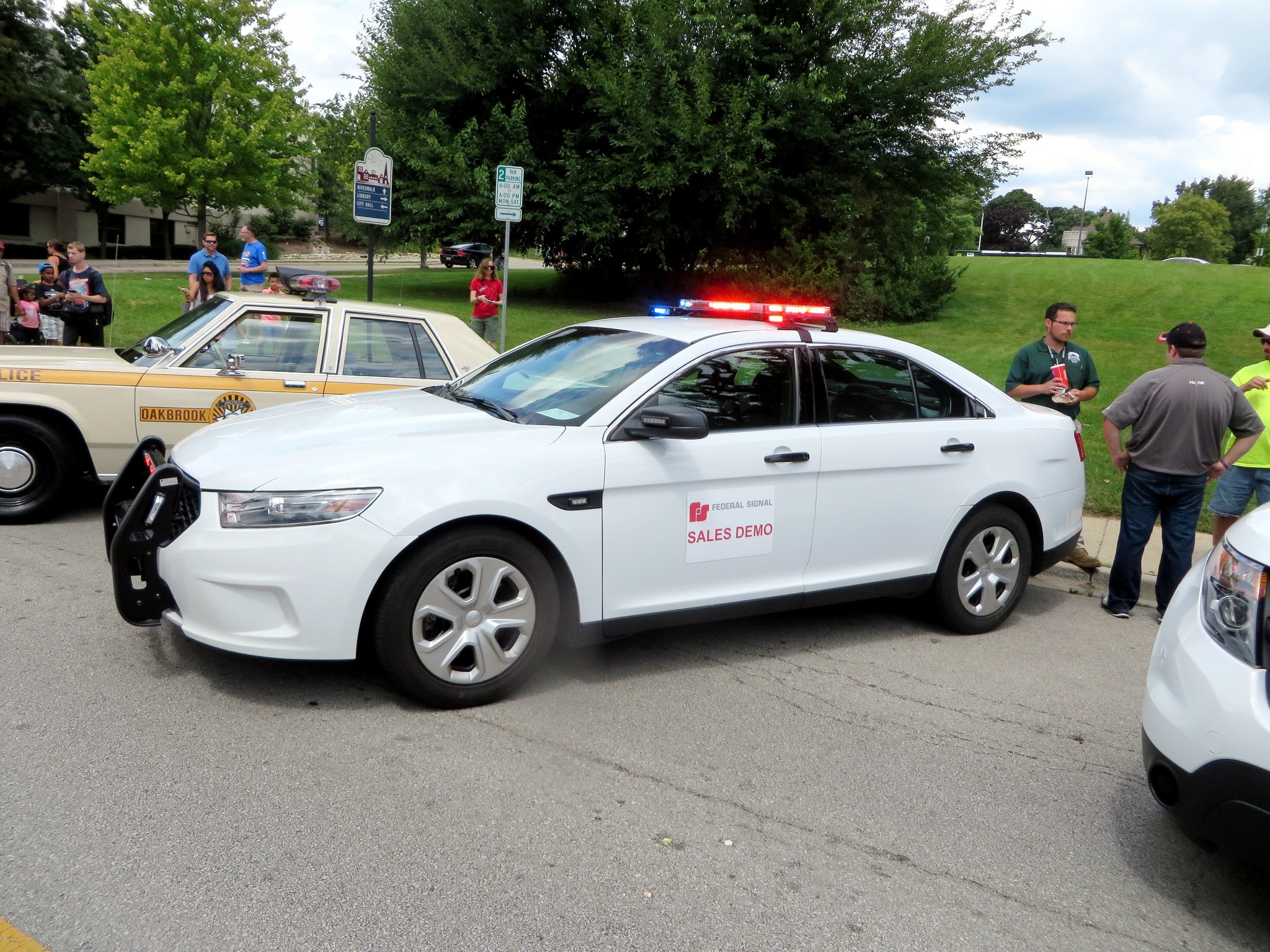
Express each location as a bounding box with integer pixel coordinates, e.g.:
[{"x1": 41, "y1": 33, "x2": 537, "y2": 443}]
[
  {"x1": 488, "y1": 300, "x2": 493, "y2": 303},
  {"x1": 69, "y1": 290, "x2": 76, "y2": 293}
]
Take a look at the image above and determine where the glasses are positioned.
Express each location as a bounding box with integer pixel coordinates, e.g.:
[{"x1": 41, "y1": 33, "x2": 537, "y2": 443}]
[
  {"x1": 201, "y1": 271, "x2": 213, "y2": 277},
  {"x1": 485, "y1": 265, "x2": 495, "y2": 270},
  {"x1": 204, "y1": 240, "x2": 218, "y2": 244},
  {"x1": 241, "y1": 231, "x2": 250, "y2": 235},
  {"x1": 46, "y1": 245, "x2": 52, "y2": 249},
  {"x1": 1048, "y1": 318, "x2": 1078, "y2": 328},
  {"x1": 1260, "y1": 338, "x2": 1270, "y2": 345}
]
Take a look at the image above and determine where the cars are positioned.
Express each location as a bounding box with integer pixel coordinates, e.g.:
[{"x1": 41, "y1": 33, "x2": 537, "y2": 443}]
[
  {"x1": 102, "y1": 300, "x2": 1088, "y2": 711},
  {"x1": 0, "y1": 265, "x2": 604, "y2": 526},
  {"x1": 1139, "y1": 498, "x2": 1269, "y2": 881},
  {"x1": 440, "y1": 242, "x2": 505, "y2": 268},
  {"x1": 1162, "y1": 257, "x2": 1211, "y2": 264}
]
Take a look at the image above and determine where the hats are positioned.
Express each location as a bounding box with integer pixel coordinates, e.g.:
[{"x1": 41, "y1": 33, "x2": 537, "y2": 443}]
[
  {"x1": 1253, "y1": 324, "x2": 1270, "y2": 338},
  {"x1": 39, "y1": 263, "x2": 53, "y2": 281},
  {"x1": 1157, "y1": 322, "x2": 1206, "y2": 349},
  {"x1": 0, "y1": 241, "x2": 5, "y2": 249}
]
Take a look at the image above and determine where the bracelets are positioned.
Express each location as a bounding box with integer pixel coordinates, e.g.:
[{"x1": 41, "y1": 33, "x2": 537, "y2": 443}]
[
  {"x1": 1219, "y1": 459, "x2": 1229, "y2": 469},
  {"x1": 476, "y1": 296, "x2": 481, "y2": 301}
]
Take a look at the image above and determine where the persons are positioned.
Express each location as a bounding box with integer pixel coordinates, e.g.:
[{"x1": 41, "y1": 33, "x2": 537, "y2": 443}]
[
  {"x1": 235, "y1": 225, "x2": 268, "y2": 345},
  {"x1": 1100, "y1": 322, "x2": 1266, "y2": 624},
  {"x1": 1206, "y1": 324, "x2": 1270, "y2": 547},
  {"x1": 0, "y1": 240, "x2": 109, "y2": 347},
  {"x1": 1006, "y1": 302, "x2": 1100, "y2": 567},
  {"x1": 187, "y1": 232, "x2": 232, "y2": 291},
  {"x1": 470, "y1": 258, "x2": 501, "y2": 350},
  {"x1": 178, "y1": 260, "x2": 225, "y2": 312},
  {"x1": 257, "y1": 272, "x2": 285, "y2": 357}
]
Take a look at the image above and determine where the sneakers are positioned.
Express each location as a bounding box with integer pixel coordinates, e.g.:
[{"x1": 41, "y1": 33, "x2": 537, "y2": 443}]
[{"x1": 1061, "y1": 547, "x2": 1101, "y2": 567}]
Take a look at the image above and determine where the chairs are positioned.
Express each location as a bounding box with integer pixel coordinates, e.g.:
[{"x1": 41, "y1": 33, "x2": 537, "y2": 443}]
[
  {"x1": 695, "y1": 359, "x2": 735, "y2": 386},
  {"x1": 736, "y1": 368, "x2": 781, "y2": 416}
]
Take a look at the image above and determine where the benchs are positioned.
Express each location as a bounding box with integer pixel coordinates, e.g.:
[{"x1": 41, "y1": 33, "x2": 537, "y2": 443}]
[{"x1": 825, "y1": 379, "x2": 940, "y2": 421}]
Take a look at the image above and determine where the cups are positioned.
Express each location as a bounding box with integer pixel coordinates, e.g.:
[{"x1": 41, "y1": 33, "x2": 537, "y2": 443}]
[
  {"x1": 63, "y1": 294, "x2": 70, "y2": 302},
  {"x1": 1050, "y1": 364, "x2": 1069, "y2": 389}
]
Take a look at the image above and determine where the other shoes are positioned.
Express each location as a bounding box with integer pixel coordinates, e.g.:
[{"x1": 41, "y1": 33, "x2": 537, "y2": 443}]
[
  {"x1": 1101, "y1": 596, "x2": 1129, "y2": 618},
  {"x1": 1158, "y1": 613, "x2": 1165, "y2": 622}
]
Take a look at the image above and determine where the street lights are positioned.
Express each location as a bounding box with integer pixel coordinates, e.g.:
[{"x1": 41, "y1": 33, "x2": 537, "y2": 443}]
[{"x1": 1076, "y1": 171, "x2": 1094, "y2": 254}]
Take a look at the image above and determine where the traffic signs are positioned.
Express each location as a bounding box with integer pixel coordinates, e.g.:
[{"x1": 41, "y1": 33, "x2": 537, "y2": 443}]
[{"x1": 352, "y1": 146, "x2": 393, "y2": 225}]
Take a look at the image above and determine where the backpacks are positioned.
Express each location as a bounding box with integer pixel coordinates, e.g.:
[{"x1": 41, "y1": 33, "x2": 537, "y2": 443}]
[{"x1": 66, "y1": 268, "x2": 115, "y2": 327}]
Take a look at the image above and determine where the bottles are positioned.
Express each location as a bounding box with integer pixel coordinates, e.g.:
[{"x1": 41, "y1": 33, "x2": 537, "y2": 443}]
[{"x1": 19, "y1": 274, "x2": 23, "y2": 279}]
